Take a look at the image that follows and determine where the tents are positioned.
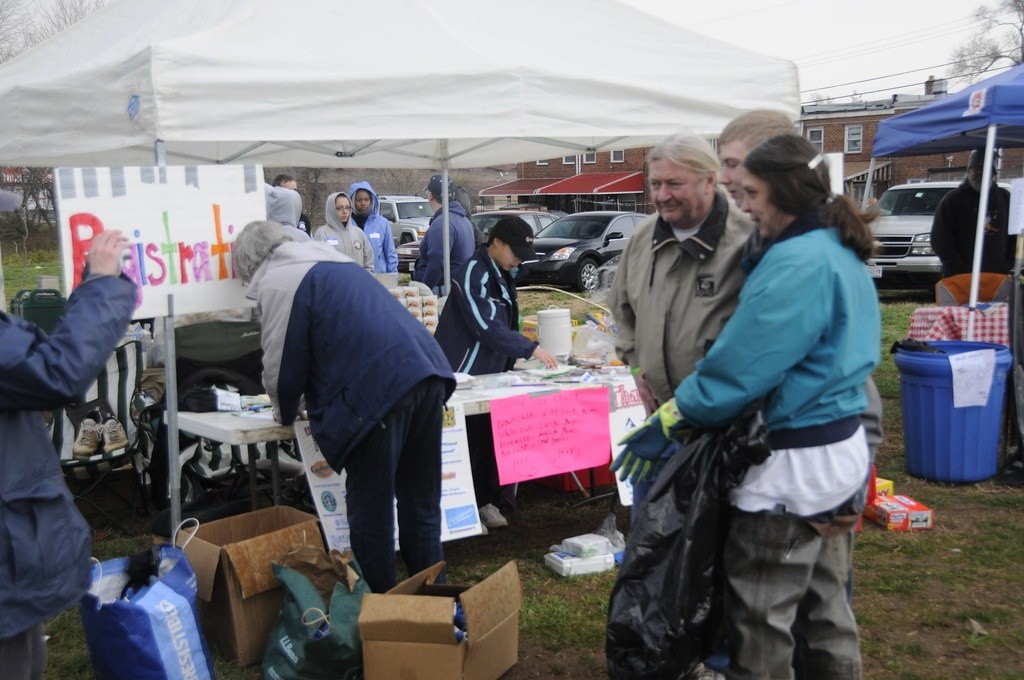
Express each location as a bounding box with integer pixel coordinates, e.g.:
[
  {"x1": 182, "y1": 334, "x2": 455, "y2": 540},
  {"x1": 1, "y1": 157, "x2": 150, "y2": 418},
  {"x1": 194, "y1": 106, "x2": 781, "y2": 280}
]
[
  {"x1": 0, "y1": 0, "x2": 804, "y2": 545},
  {"x1": 861, "y1": 61, "x2": 1024, "y2": 342}
]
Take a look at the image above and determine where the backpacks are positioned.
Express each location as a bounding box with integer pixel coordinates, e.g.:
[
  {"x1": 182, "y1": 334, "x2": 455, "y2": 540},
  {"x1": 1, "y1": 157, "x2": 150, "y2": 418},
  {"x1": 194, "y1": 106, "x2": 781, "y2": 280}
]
[{"x1": 147, "y1": 365, "x2": 281, "y2": 511}]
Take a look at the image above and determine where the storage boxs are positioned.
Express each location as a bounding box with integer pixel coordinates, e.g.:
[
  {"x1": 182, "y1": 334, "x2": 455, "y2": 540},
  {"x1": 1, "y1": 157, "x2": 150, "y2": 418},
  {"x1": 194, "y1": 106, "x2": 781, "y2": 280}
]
[
  {"x1": 357, "y1": 560, "x2": 521, "y2": 680},
  {"x1": 863, "y1": 477, "x2": 931, "y2": 531},
  {"x1": 545, "y1": 551, "x2": 616, "y2": 578},
  {"x1": 175, "y1": 504, "x2": 323, "y2": 664}
]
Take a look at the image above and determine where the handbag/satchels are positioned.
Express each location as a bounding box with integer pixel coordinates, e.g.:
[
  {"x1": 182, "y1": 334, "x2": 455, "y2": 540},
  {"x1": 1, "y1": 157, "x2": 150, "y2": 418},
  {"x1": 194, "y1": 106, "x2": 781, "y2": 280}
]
[
  {"x1": 260, "y1": 535, "x2": 374, "y2": 680},
  {"x1": 75, "y1": 515, "x2": 213, "y2": 680}
]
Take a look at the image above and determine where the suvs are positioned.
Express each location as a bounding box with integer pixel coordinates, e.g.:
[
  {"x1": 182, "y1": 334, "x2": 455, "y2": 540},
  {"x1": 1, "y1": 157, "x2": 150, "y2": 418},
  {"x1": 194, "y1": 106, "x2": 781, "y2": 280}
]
[{"x1": 376, "y1": 194, "x2": 436, "y2": 247}]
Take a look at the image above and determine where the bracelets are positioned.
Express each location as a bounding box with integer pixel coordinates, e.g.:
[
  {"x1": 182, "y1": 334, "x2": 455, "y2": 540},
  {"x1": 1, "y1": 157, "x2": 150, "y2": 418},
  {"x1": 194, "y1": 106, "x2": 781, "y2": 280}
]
[{"x1": 631, "y1": 367, "x2": 641, "y2": 377}]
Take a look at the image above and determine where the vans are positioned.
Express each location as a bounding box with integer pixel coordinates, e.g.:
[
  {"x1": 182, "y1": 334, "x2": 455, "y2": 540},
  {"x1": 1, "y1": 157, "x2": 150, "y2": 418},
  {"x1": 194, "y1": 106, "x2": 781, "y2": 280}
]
[{"x1": 867, "y1": 179, "x2": 1011, "y2": 289}]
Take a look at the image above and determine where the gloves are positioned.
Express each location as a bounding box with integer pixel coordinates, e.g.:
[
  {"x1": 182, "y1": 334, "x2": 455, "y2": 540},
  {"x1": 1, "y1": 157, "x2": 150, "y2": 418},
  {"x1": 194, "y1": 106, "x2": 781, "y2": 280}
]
[{"x1": 610, "y1": 401, "x2": 693, "y2": 487}]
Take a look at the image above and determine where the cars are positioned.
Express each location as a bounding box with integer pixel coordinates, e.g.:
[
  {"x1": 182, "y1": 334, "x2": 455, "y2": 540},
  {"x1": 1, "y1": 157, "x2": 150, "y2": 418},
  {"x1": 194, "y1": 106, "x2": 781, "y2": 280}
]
[
  {"x1": 395, "y1": 208, "x2": 569, "y2": 274},
  {"x1": 514, "y1": 210, "x2": 650, "y2": 293}
]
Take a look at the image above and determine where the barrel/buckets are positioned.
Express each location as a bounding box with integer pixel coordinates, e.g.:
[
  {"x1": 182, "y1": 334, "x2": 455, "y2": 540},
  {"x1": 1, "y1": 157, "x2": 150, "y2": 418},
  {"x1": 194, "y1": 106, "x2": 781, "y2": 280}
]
[{"x1": 536, "y1": 305, "x2": 572, "y2": 356}]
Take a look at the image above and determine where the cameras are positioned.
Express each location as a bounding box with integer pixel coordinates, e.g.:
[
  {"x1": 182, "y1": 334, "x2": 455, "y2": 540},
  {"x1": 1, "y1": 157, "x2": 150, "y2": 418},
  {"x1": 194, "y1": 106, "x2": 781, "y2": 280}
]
[{"x1": 119, "y1": 247, "x2": 133, "y2": 270}]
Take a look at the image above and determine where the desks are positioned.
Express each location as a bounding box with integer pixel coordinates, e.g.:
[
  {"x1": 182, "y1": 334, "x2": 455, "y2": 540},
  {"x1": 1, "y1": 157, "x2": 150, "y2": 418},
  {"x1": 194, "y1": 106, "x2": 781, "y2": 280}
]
[
  {"x1": 167, "y1": 365, "x2": 639, "y2": 518},
  {"x1": 911, "y1": 304, "x2": 1009, "y2": 353}
]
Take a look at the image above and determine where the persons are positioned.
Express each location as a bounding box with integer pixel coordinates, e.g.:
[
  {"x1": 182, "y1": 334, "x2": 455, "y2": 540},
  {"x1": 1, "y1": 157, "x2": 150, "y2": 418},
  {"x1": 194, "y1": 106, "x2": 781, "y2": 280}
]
[
  {"x1": 413, "y1": 175, "x2": 475, "y2": 321},
  {"x1": 718, "y1": 110, "x2": 885, "y2": 607},
  {"x1": 612, "y1": 132, "x2": 882, "y2": 679},
  {"x1": 929, "y1": 148, "x2": 1017, "y2": 277},
  {"x1": 607, "y1": 130, "x2": 757, "y2": 680},
  {"x1": 234, "y1": 219, "x2": 457, "y2": 594},
  {"x1": 0, "y1": 227, "x2": 141, "y2": 680},
  {"x1": 267, "y1": 174, "x2": 310, "y2": 239},
  {"x1": 454, "y1": 184, "x2": 488, "y2": 252},
  {"x1": 347, "y1": 181, "x2": 398, "y2": 274},
  {"x1": 314, "y1": 191, "x2": 374, "y2": 273},
  {"x1": 434, "y1": 216, "x2": 559, "y2": 535}
]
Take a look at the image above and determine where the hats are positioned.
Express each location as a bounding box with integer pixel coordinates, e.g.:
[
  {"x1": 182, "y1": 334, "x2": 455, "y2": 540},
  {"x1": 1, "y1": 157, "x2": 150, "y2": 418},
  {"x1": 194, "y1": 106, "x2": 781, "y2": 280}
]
[
  {"x1": 490, "y1": 217, "x2": 537, "y2": 261},
  {"x1": 967, "y1": 150, "x2": 999, "y2": 175},
  {"x1": 423, "y1": 175, "x2": 456, "y2": 200}
]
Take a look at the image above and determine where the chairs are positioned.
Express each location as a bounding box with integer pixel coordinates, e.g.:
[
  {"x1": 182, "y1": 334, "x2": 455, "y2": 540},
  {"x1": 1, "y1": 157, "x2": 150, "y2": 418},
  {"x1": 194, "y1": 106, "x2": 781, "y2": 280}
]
[
  {"x1": 935, "y1": 273, "x2": 1009, "y2": 309},
  {"x1": 48, "y1": 341, "x2": 150, "y2": 535}
]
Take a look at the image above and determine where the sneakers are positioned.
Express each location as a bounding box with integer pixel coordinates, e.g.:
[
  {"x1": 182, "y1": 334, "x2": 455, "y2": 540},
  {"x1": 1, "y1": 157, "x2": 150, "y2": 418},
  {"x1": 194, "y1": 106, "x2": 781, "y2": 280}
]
[
  {"x1": 100, "y1": 416, "x2": 128, "y2": 452},
  {"x1": 74, "y1": 410, "x2": 103, "y2": 457}
]
